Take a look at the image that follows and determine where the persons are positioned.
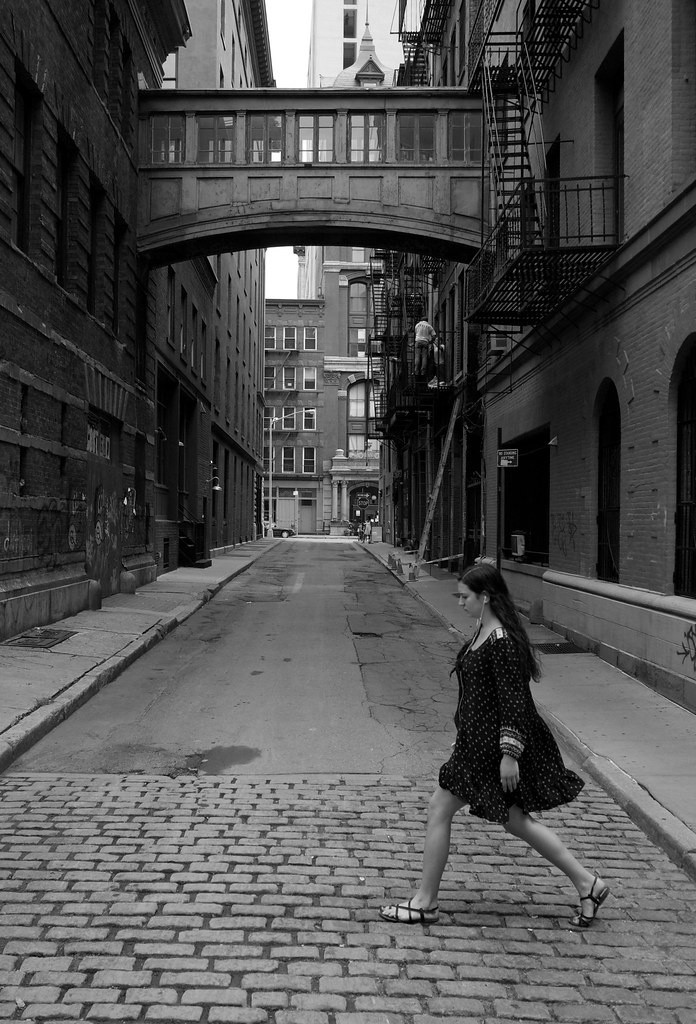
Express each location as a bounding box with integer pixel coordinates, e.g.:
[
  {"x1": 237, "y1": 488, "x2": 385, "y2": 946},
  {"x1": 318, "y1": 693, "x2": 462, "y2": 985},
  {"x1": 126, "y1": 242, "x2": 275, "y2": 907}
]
[
  {"x1": 412, "y1": 316, "x2": 436, "y2": 376},
  {"x1": 363, "y1": 518, "x2": 371, "y2": 544},
  {"x1": 376, "y1": 563, "x2": 611, "y2": 926},
  {"x1": 432, "y1": 336, "x2": 448, "y2": 386},
  {"x1": 357, "y1": 520, "x2": 363, "y2": 540}
]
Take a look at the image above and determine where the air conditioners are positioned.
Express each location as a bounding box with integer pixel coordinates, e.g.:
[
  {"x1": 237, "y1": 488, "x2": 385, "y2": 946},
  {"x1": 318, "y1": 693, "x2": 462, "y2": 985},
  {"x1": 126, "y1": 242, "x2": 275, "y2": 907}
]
[
  {"x1": 488, "y1": 338, "x2": 507, "y2": 355},
  {"x1": 511, "y1": 535, "x2": 525, "y2": 556}
]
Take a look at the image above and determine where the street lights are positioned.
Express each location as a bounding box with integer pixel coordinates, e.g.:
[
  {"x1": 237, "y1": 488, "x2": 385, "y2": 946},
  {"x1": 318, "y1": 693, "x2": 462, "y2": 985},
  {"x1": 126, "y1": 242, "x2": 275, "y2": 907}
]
[{"x1": 267, "y1": 410, "x2": 316, "y2": 538}]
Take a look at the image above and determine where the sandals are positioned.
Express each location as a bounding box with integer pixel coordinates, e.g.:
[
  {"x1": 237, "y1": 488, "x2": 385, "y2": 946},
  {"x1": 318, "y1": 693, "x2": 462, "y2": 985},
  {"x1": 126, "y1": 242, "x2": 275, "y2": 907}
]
[
  {"x1": 378, "y1": 899, "x2": 439, "y2": 922},
  {"x1": 569, "y1": 877, "x2": 610, "y2": 927}
]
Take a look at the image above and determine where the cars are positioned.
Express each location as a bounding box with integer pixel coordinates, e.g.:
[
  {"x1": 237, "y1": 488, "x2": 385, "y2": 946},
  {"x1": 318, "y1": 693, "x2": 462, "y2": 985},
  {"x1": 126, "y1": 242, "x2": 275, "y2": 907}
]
[{"x1": 265, "y1": 523, "x2": 297, "y2": 538}]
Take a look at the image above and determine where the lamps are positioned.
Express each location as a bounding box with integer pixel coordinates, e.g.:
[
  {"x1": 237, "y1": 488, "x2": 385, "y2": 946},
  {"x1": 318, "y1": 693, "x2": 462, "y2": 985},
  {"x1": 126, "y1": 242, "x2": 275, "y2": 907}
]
[{"x1": 206, "y1": 476, "x2": 223, "y2": 491}]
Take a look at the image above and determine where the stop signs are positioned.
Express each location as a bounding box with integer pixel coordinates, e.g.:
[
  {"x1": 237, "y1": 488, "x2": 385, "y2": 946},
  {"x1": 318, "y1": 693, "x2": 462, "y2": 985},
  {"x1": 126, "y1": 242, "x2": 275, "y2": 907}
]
[{"x1": 357, "y1": 498, "x2": 369, "y2": 510}]
